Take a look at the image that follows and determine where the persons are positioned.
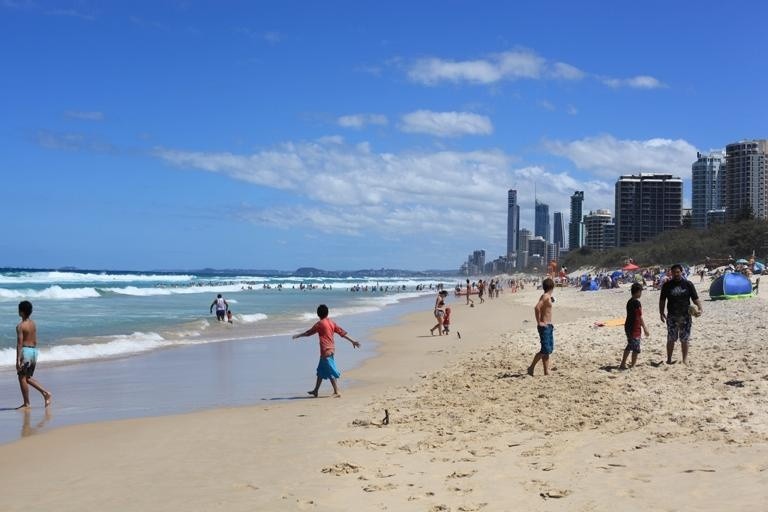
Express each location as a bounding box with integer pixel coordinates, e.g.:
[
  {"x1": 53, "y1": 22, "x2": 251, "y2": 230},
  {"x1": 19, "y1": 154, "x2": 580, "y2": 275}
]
[
  {"x1": 430, "y1": 290, "x2": 450, "y2": 336},
  {"x1": 291, "y1": 304, "x2": 361, "y2": 399},
  {"x1": 224, "y1": 309, "x2": 233, "y2": 324},
  {"x1": 13, "y1": 300, "x2": 52, "y2": 410},
  {"x1": 618, "y1": 282, "x2": 651, "y2": 371},
  {"x1": 658, "y1": 264, "x2": 702, "y2": 368},
  {"x1": 526, "y1": 279, "x2": 557, "y2": 377},
  {"x1": 442, "y1": 308, "x2": 452, "y2": 336},
  {"x1": 210, "y1": 294, "x2": 228, "y2": 322}
]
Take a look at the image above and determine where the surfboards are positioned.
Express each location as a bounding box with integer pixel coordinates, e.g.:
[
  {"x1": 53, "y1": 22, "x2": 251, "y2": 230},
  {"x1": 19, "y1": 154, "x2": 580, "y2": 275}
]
[{"x1": 593, "y1": 319, "x2": 627, "y2": 326}]
